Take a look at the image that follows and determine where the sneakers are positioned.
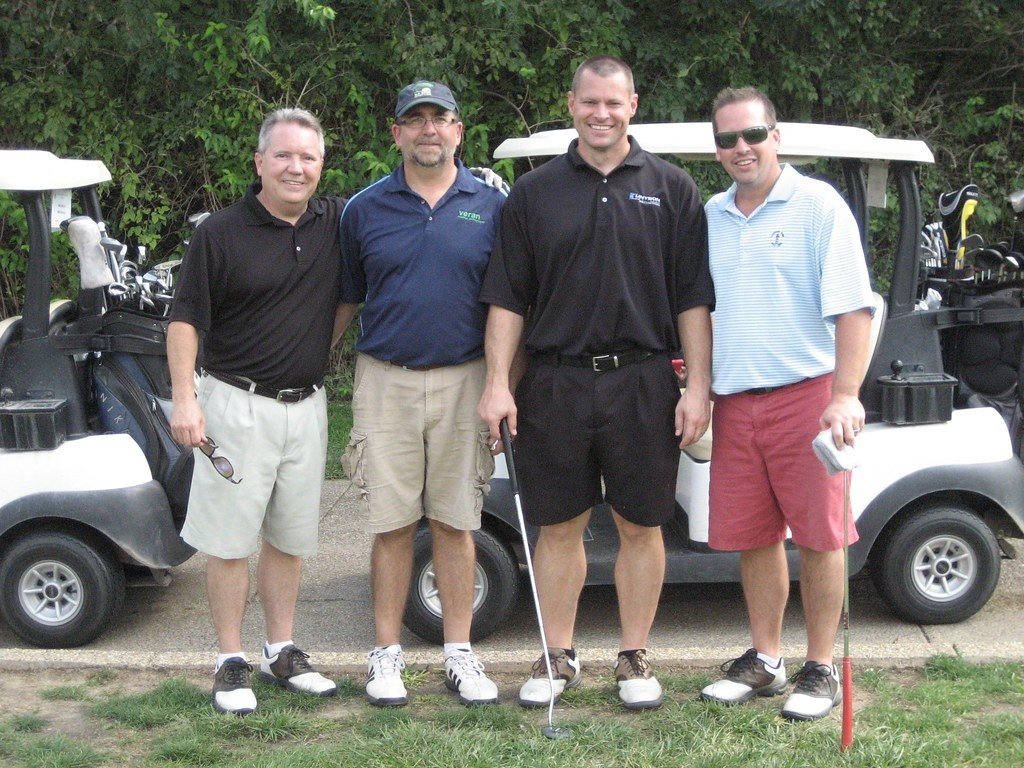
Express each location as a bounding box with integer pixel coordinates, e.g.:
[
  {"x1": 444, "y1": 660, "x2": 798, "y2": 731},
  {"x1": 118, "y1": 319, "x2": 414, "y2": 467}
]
[
  {"x1": 783, "y1": 660, "x2": 842, "y2": 720},
  {"x1": 613, "y1": 650, "x2": 662, "y2": 709},
  {"x1": 700, "y1": 648, "x2": 789, "y2": 706},
  {"x1": 260, "y1": 644, "x2": 336, "y2": 697},
  {"x1": 212, "y1": 656, "x2": 257, "y2": 716},
  {"x1": 366, "y1": 644, "x2": 408, "y2": 705},
  {"x1": 444, "y1": 648, "x2": 498, "y2": 707},
  {"x1": 520, "y1": 646, "x2": 581, "y2": 708}
]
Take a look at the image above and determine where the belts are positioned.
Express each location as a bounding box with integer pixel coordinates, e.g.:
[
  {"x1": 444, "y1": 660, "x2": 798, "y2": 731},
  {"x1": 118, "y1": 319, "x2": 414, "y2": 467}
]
[
  {"x1": 206, "y1": 370, "x2": 325, "y2": 403},
  {"x1": 542, "y1": 347, "x2": 658, "y2": 372},
  {"x1": 745, "y1": 375, "x2": 812, "y2": 396}
]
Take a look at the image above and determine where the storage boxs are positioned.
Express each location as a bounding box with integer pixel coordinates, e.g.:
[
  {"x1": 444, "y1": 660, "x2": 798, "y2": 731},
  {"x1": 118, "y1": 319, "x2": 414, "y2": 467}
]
[
  {"x1": 880, "y1": 372, "x2": 959, "y2": 423},
  {"x1": 0, "y1": 395, "x2": 67, "y2": 452}
]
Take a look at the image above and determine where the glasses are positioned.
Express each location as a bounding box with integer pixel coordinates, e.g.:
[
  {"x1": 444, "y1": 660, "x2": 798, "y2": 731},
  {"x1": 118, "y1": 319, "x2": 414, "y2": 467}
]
[
  {"x1": 199, "y1": 436, "x2": 243, "y2": 485},
  {"x1": 397, "y1": 116, "x2": 457, "y2": 129},
  {"x1": 714, "y1": 123, "x2": 776, "y2": 149}
]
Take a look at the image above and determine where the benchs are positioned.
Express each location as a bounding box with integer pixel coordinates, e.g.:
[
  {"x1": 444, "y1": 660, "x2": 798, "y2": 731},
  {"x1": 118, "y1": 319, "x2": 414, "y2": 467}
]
[
  {"x1": 675, "y1": 290, "x2": 885, "y2": 552},
  {"x1": 0, "y1": 298, "x2": 77, "y2": 359}
]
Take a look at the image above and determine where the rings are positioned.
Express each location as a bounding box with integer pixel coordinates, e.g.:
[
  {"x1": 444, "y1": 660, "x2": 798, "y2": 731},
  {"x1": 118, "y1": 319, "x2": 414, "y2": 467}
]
[{"x1": 853, "y1": 428, "x2": 860, "y2": 431}]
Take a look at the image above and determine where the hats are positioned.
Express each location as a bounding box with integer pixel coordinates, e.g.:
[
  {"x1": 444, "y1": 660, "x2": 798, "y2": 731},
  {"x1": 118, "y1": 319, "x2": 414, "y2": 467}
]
[{"x1": 394, "y1": 80, "x2": 459, "y2": 118}]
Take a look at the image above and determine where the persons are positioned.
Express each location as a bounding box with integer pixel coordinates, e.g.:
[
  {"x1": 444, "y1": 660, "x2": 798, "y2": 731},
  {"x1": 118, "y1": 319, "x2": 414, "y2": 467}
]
[
  {"x1": 166, "y1": 108, "x2": 512, "y2": 716},
  {"x1": 479, "y1": 56, "x2": 717, "y2": 708},
  {"x1": 328, "y1": 82, "x2": 513, "y2": 706},
  {"x1": 702, "y1": 85, "x2": 875, "y2": 719}
]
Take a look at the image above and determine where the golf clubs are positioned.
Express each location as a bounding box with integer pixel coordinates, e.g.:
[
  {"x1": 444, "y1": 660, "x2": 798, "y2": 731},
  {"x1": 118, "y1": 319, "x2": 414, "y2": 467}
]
[
  {"x1": 99, "y1": 209, "x2": 211, "y2": 315},
  {"x1": 839, "y1": 468, "x2": 854, "y2": 754},
  {"x1": 499, "y1": 416, "x2": 573, "y2": 740},
  {"x1": 920, "y1": 190, "x2": 1024, "y2": 283}
]
